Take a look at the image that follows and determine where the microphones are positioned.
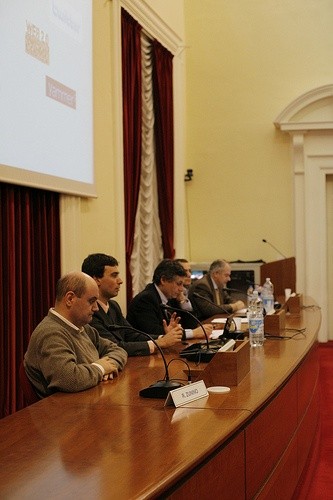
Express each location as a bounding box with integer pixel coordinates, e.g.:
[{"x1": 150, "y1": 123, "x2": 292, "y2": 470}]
[
  {"x1": 160, "y1": 304, "x2": 217, "y2": 363},
  {"x1": 188, "y1": 291, "x2": 244, "y2": 343},
  {"x1": 220, "y1": 288, "x2": 267, "y2": 318},
  {"x1": 262, "y1": 239, "x2": 287, "y2": 259},
  {"x1": 108, "y1": 324, "x2": 181, "y2": 400}
]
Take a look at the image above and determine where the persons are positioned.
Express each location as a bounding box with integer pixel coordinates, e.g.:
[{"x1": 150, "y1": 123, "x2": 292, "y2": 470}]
[
  {"x1": 188, "y1": 258, "x2": 244, "y2": 321},
  {"x1": 126, "y1": 259, "x2": 213, "y2": 339},
  {"x1": 24, "y1": 271, "x2": 128, "y2": 400},
  {"x1": 83, "y1": 253, "x2": 183, "y2": 356},
  {"x1": 166, "y1": 259, "x2": 204, "y2": 323}
]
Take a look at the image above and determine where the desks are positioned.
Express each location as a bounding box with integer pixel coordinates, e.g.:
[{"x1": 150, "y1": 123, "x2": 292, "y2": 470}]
[{"x1": 0, "y1": 292, "x2": 333, "y2": 500}]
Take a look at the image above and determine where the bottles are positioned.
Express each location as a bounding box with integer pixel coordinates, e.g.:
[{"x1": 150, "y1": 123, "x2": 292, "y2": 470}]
[
  {"x1": 248, "y1": 284, "x2": 264, "y2": 348},
  {"x1": 264, "y1": 278, "x2": 275, "y2": 315}
]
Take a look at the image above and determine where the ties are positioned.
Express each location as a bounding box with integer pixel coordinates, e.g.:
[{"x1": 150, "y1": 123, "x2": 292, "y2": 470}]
[
  {"x1": 166, "y1": 302, "x2": 172, "y2": 319},
  {"x1": 216, "y1": 288, "x2": 220, "y2": 306},
  {"x1": 107, "y1": 308, "x2": 111, "y2": 319}
]
